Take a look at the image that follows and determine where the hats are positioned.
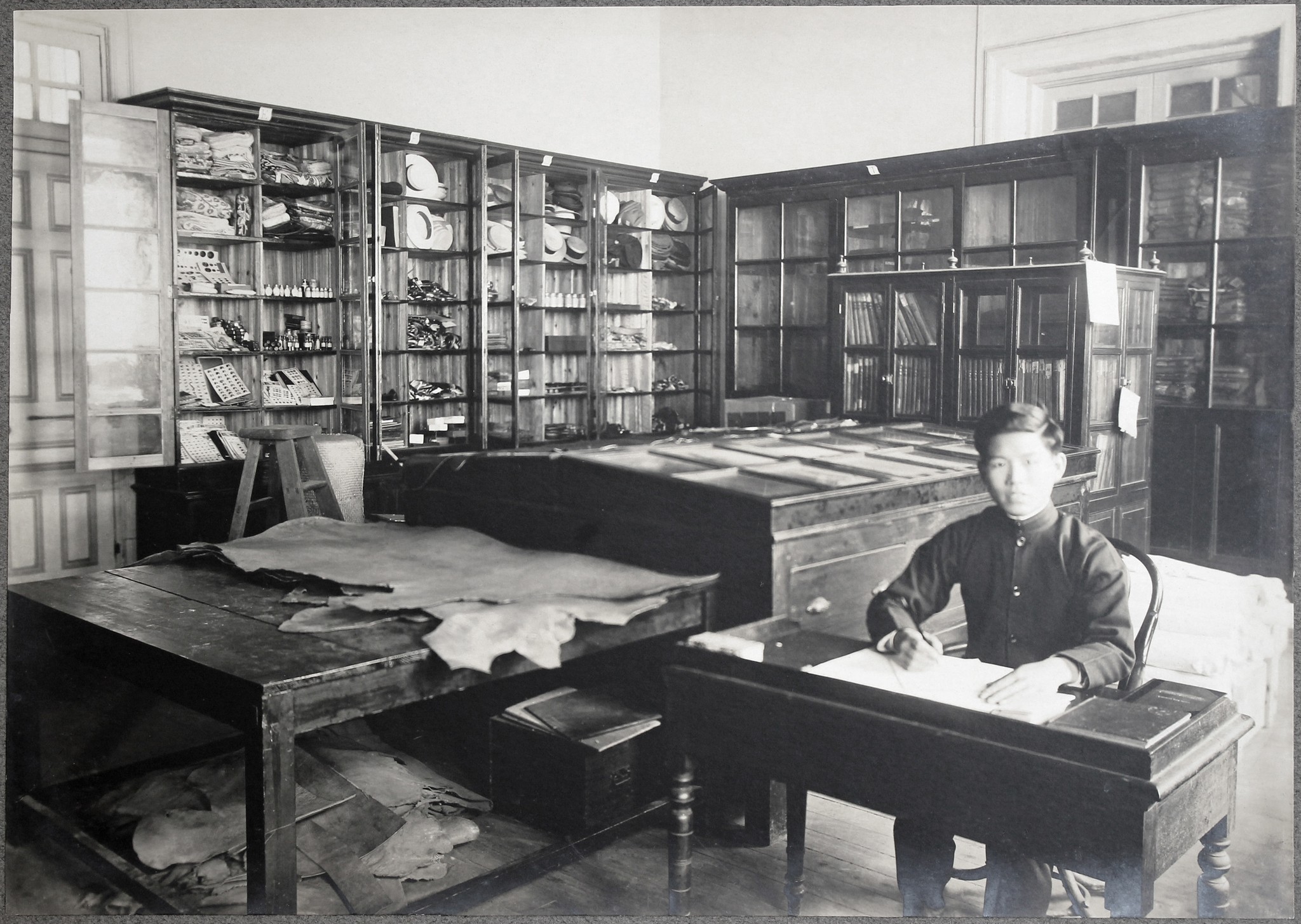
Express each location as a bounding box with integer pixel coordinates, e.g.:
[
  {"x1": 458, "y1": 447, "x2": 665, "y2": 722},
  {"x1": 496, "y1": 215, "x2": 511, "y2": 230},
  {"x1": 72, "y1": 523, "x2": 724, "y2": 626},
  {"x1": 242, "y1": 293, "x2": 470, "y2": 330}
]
[
  {"x1": 487, "y1": 181, "x2": 521, "y2": 213},
  {"x1": 652, "y1": 193, "x2": 691, "y2": 232},
  {"x1": 606, "y1": 235, "x2": 643, "y2": 268},
  {"x1": 545, "y1": 179, "x2": 584, "y2": 220},
  {"x1": 651, "y1": 234, "x2": 694, "y2": 271},
  {"x1": 485, "y1": 219, "x2": 528, "y2": 261},
  {"x1": 402, "y1": 204, "x2": 455, "y2": 252},
  {"x1": 405, "y1": 154, "x2": 449, "y2": 203},
  {"x1": 606, "y1": 188, "x2": 644, "y2": 227},
  {"x1": 544, "y1": 222, "x2": 588, "y2": 265}
]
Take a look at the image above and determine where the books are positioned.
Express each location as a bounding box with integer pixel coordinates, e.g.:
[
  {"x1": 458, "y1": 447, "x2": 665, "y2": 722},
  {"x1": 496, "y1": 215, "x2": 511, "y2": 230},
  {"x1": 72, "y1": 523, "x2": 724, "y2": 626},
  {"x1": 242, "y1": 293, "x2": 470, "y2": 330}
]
[
  {"x1": 1046, "y1": 695, "x2": 1191, "y2": 750},
  {"x1": 1158, "y1": 280, "x2": 1248, "y2": 324},
  {"x1": 845, "y1": 354, "x2": 1065, "y2": 420},
  {"x1": 368, "y1": 414, "x2": 407, "y2": 450},
  {"x1": 847, "y1": 291, "x2": 939, "y2": 346},
  {"x1": 1151, "y1": 356, "x2": 1252, "y2": 409},
  {"x1": 502, "y1": 685, "x2": 662, "y2": 753}
]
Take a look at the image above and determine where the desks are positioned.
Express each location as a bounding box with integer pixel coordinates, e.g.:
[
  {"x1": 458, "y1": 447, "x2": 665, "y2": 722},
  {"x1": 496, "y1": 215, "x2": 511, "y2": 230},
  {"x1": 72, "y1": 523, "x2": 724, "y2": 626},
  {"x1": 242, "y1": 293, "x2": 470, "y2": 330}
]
[{"x1": 3, "y1": 522, "x2": 1242, "y2": 915}]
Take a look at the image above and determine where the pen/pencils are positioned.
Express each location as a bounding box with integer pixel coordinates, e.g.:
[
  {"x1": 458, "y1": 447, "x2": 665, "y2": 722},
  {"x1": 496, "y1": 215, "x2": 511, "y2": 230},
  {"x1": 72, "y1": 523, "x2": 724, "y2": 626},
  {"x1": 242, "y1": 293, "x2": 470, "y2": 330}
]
[{"x1": 900, "y1": 595, "x2": 925, "y2": 639}]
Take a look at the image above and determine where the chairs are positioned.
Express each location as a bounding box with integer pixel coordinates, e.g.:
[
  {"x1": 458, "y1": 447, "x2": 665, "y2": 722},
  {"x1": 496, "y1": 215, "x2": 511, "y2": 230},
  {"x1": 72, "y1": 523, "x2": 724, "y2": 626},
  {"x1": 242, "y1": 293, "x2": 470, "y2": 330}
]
[{"x1": 1110, "y1": 536, "x2": 1164, "y2": 690}]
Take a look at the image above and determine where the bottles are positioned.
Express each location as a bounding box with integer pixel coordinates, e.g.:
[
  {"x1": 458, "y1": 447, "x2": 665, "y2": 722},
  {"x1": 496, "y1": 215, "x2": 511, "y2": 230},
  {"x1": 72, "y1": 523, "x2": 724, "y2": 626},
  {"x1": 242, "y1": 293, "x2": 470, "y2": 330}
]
[
  {"x1": 545, "y1": 293, "x2": 586, "y2": 307},
  {"x1": 263, "y1": 278, "x2": 333, "y2": 298},
  {"x1": 263, "y1": 314, "x2": 332, "y2": 351}
]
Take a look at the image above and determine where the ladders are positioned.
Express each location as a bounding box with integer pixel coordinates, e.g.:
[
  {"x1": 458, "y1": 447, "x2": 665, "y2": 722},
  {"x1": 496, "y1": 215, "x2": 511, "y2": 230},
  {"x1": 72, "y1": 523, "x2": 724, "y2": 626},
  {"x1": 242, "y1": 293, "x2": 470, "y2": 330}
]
[{"x1": 229, "y1": 424, "x2": 345, "y2": 537}]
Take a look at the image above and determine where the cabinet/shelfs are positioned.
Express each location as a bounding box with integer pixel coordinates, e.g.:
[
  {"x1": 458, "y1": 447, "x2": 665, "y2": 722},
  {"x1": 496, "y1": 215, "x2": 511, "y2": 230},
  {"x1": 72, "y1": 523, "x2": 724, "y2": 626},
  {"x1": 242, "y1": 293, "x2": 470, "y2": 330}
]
[{"x1": 69, "y1": 88, "x2": 1301, "y2": 582}]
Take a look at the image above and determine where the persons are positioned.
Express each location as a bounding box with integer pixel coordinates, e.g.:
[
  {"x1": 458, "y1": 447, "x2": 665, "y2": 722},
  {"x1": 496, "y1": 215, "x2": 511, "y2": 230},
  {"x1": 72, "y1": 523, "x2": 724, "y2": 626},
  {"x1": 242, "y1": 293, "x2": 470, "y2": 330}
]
[{"x1": 865, "y1": 404, "x2": 1135, "y2": 916}]
